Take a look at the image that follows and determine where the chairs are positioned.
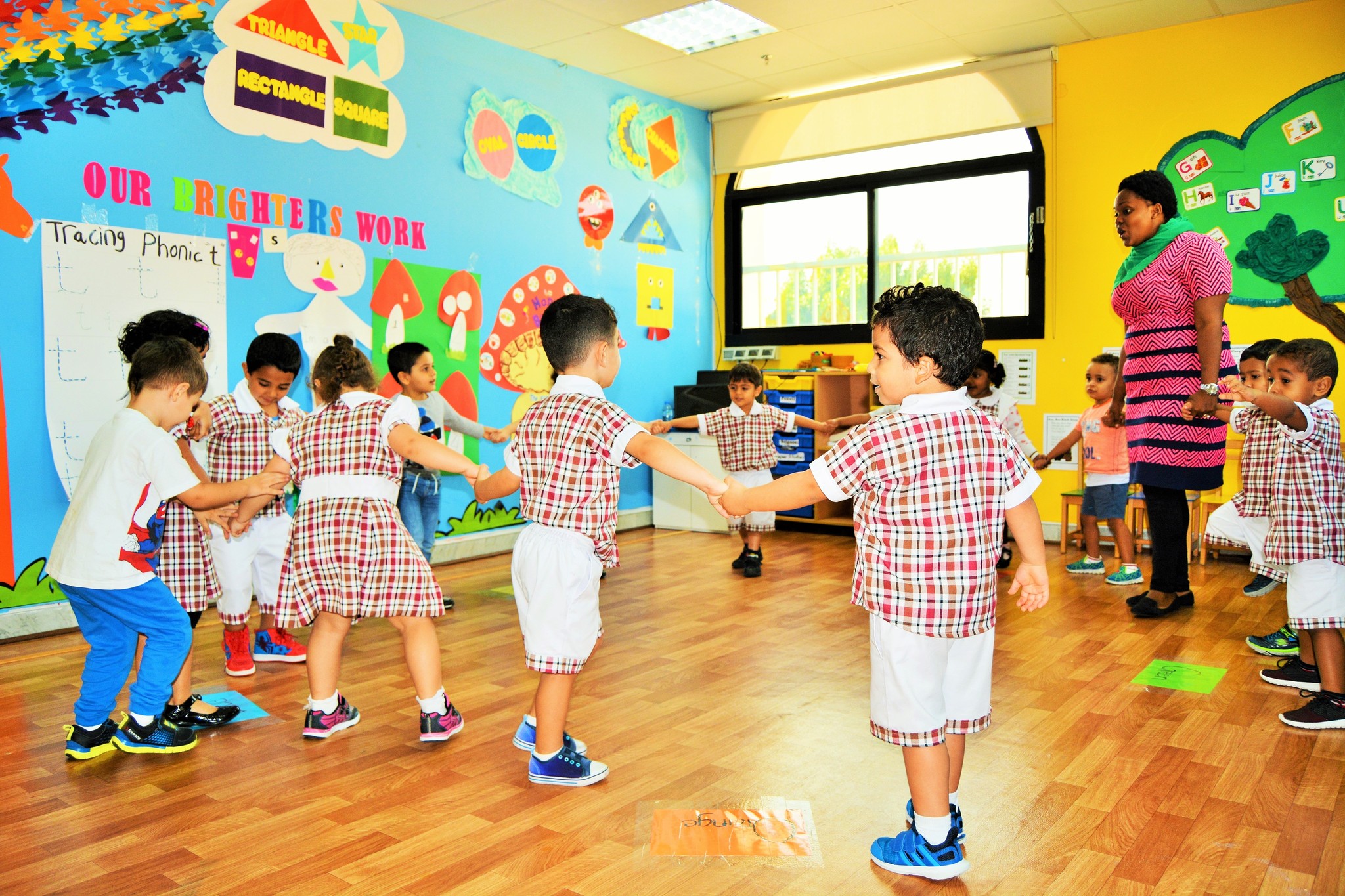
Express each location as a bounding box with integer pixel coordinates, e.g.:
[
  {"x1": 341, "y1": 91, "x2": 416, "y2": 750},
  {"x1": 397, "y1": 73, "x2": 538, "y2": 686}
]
[{"x1": 1048, "y1": 432, "x2": 1279, "y2": 570}]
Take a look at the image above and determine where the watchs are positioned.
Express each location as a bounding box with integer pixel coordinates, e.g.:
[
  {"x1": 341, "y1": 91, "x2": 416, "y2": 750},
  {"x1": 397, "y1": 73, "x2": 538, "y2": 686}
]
[{"x1": 1198, "y1": 383, "x2": 1219, "y2": 396}]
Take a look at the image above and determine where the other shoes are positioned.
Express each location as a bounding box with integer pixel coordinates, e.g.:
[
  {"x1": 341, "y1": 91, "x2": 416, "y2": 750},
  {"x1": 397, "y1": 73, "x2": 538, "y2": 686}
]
[
  {"x1": 1130, "y1": 592, "x2": 1181, "y2": 617},
  {"x1": 1126, "y1": 590, "x2": 1195, "y2": 607}
]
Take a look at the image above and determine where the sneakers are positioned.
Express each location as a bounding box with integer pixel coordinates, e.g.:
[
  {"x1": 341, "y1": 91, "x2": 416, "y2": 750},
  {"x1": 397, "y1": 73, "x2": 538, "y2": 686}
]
[
  {"x1": 731, "y1": 543, "x2": 763, "y2": 577},
  {"x1": 112, "y1": 711, "x2": 198, "y2": 754},
  {"x1": 870, "y1": 813, "x2": 970, "y2": 880},
  {"x1": 302, "y1": 692, "x2": 361, "y2": 739},
  {"x1": 1246, "y1": 622, "x2": 1301, "y2": 657},
  {"x1": 419, "y1": 693, "x2": 464, "y2": 742},
  {"x1": 1104, "y1": 563, "x2": 1144, "y2": 584},
  {"x1": 1242, "y1": 572, "x2": 1279, "y2": 597},
  {"x1": 221, "y1": 622, "x2": 256, "y2": 677},
  {"x1": 1259, "y1": 655, "x2": 1321, "y2": 692},
  {"x1": 442, "y1": 595, "x2": 455, "y2": 609},
  {"x1": 1278, "y1": 691, "x2": 1345, "y2": 729},
  {"x1": 511, "y1": 714, "x2": 610, "y2": 787},
  {"x1": 251, "y1": 626, "x2": 307, "y2": 664},
  {"x1": 62, "y1": 718, "x2": 120, "y2": 760},
  {"x1": 905, "y1": 798, "x2": 967, "y2": 845},
  {"x1": 1065, "y1": 554, "x2": 1106, "y2": 574}
]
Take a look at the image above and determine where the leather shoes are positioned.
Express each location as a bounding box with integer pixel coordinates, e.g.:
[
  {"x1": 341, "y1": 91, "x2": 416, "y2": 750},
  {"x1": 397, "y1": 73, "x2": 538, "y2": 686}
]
[
  {"x1": 995, "y1": 547, "x2": 1012, "y2": 569},
  {"x1": 162, "y1": 694, "x2": 241, "y2": 729}
]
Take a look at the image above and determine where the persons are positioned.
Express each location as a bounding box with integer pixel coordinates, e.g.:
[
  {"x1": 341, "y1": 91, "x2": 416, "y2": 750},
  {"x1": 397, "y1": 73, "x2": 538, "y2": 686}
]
[
  {"x1": 720, "y1": 282, "x2": 1052, "y2": 887},
  {"x1": 1215, "y1": 335, "x2": 1345, "y2": 732},
  {"x1": 1034, "y1": 353, "x2": 1148, "y2": 588},
  {"x1": 1181, "y1": 338, "x2": 1302, "y2": 657},
  {"x1": 487, "y1": 367, "x2": 666, "y2": 580},
  {"x1": 650, "y1": 362, "x2": 838, "y2": 579},
  {"x1": 115, "y1": 306, "x2": 243, "y2": 728},
  {"x1": 825, "y1": 404, "x2": 901, "y2": 433},
  {"x1": 477, "y1": 291, "x2": 746, "y2": 791},
  {"x1": 227, "y1": 335, "x2": 491, "y2": 746},
  {"x1": 44, "y1": 334, "x2": 294, "y2": 765},
  {"x1": 384, "y1": 341, "x2": 505, "y2": 614},
  {"x1": 965, "y1": 348, "x2": 1050, "y2": 568},
  {"x1": 192, "y1": 329, "x2": 309, "y2": 678},
  {"x1": 1108, "y1": 168, "x2": 1240, "y2": 619}
]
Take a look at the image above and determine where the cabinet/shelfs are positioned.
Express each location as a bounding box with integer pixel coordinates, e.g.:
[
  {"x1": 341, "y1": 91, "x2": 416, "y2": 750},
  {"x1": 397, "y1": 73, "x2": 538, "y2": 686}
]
[{"x1": 647, "y1": 366, "x2": 940, "y2": 538}]
